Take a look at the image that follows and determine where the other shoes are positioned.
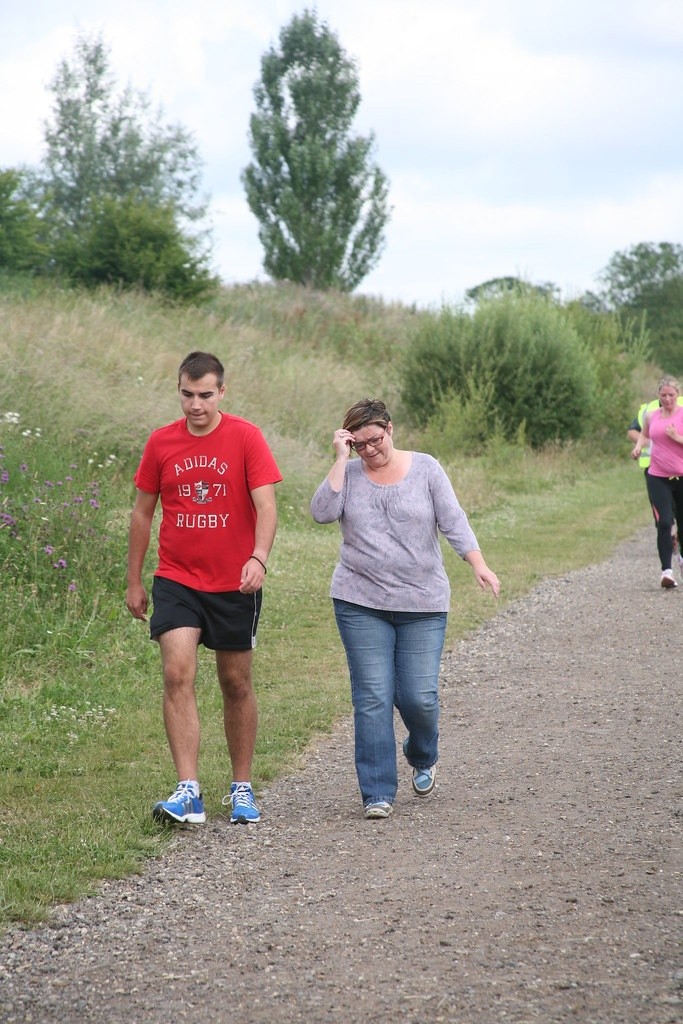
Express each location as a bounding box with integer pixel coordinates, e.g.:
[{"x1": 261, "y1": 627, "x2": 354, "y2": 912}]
[
  {"x1": 660, "y1": 569, "x2": 678, "y2": 590},
  {"x1": 678, "y1": 554, "x2": 683, "y2": 578}
]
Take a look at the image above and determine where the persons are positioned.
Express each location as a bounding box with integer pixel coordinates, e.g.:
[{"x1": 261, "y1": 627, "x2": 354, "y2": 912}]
[
  {"x1": 627, "y1": 375, "x2": 683, "y2": 588},
  {"x1": 309, "y1": 399, "x2": 502, "y2": 818},
  {"x1": 126, "y1": 352, "x2": 283, "y2": 824}
]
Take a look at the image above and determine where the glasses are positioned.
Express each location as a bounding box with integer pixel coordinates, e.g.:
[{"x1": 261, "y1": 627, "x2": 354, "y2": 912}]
[{"x1": 348, "y1": 427, "x2": 388, "y2": 451}]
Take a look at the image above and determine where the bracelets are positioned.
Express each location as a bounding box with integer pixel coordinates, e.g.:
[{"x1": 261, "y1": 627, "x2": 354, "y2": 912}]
[{"x1": 251, "y1": 556, "x2": 267, "y2": 574}]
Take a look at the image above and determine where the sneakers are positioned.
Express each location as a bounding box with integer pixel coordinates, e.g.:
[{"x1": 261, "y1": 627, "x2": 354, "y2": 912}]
[
  {"x1": 363, "y1": 801, "x2": 395, "y2": 819},
  {"x1": 222, "y1": 782, "x2": 260, "y2": 824},
  {"x1": 152, "y1": 778, "x2": 207, "y2": 823},
  {"x1": 411, "y1": 764, "x2": 436, "y2": 799}
]
